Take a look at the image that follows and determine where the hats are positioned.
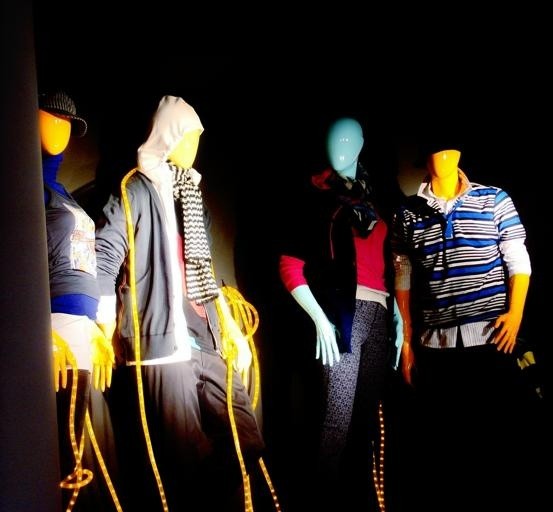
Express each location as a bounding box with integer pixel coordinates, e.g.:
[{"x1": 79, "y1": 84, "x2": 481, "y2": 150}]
[{"x1": 35, "y1": 91, "x2": 87, "y2": 139}]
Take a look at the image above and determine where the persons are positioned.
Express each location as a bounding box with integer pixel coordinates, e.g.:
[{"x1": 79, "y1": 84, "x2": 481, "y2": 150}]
[
  {"x1": 39, "y1": 86, "x2": 119, "y2": 511},
  {"x1": 93, "y1": 93, "x2": 266, "y2": 481},
  {"x1": 393, "y1": 146, "x2": 531, "y2": 385},
  {"x1": 276, "y1": 116, "x2": 402, "y2": 461}
]
